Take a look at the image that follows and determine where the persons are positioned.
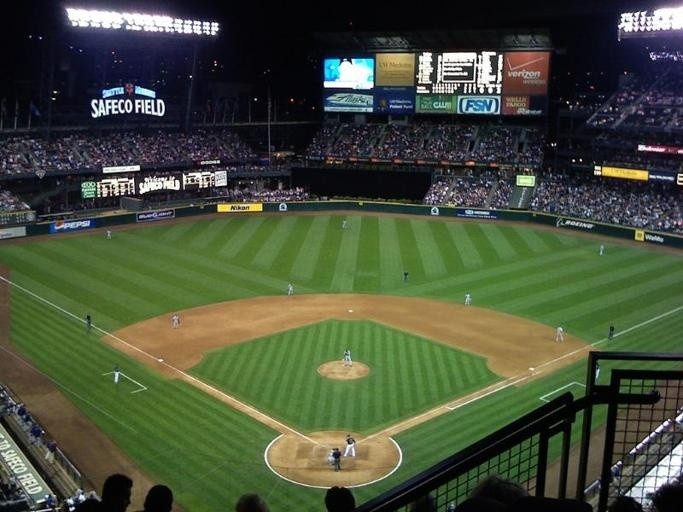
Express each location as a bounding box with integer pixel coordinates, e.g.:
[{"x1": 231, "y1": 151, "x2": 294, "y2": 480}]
[
  {"x1": 464, "y1": 292, "x2": 470, "y2": 308},
  {"x1": 86, "y1": 314, "x2": 92, "y2": 327},
  {"x1": 1, "y1": 388, "x2": 56, "y2": 464},
  {"x1": 239, "y1": 494, "x2": 267, "y2": 511},
  {"x1": 344, "y1": 349, "x2": 352, "y2": 367},
  {"x1": 114, "y1": 367, "x2": 120, "y2": 383},
  {"x1": 0, "y1": 67, "x2": 682, "y2": 235},
  {"x1": 609, "y1": 324, "x2": 614, "y2": 339},
  {"x1": 412, "y1": 475, "x2": 682, "y2": 512},
  {"x1": 107, "y1": 229, "x2": 112, "y2": 241},
  {"x1": 287, "y1": 284, "x2": 293, "y2": 296},
  {"x1": 600, "y1": 244, "x2": 604, "y2": 255},
  {"x1": 555, "y1": 326, "x2": 564, "y2": 342},
  {"x1": 0, "y1": 472, "x2": 173, "y2": 511},
  {"x1": 328, "y1": 434, "x2": 356, "y2": 471},
  {"x1": 403, "y1": 271, "x2": 408, "y2": 281},
  {"x1": 172, "y1": 314, "x2": 180, "y2": 329},
  {"x1": 325, "y1": 486, "x2": 356, "y2": 511}
]
